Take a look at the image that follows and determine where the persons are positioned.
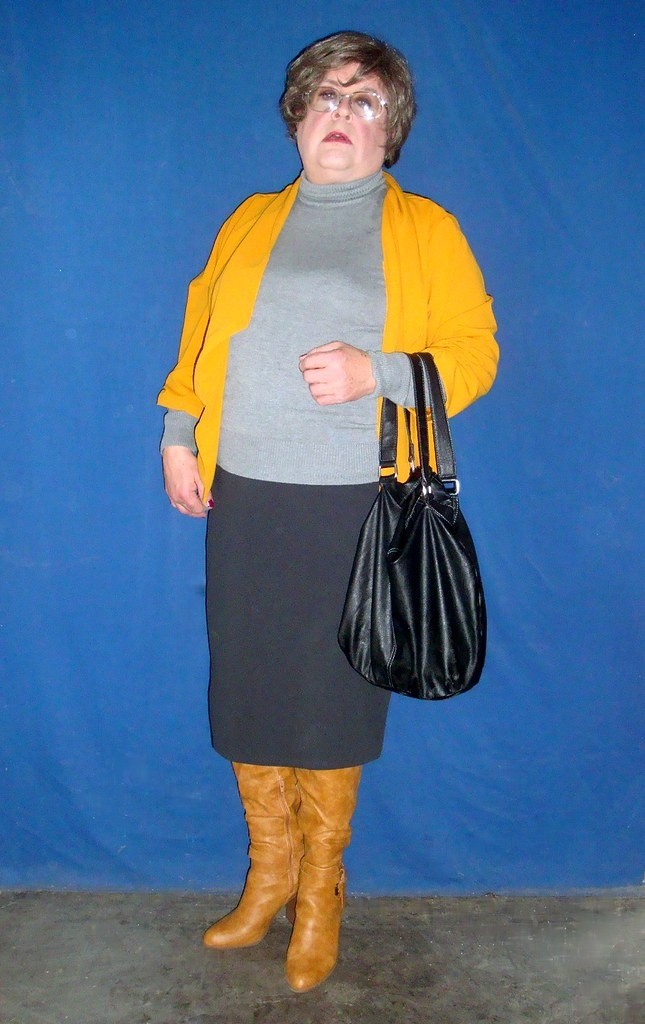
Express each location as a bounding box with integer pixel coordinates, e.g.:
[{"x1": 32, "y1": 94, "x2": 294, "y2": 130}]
[{"x1": 157, "y1": 30, "x2": 499, "y2": 993}]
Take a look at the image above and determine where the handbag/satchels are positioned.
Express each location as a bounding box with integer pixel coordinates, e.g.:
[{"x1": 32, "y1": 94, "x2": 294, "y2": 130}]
[{"x1": 336, "y1": 352, "x2": 488, "y2": 700}]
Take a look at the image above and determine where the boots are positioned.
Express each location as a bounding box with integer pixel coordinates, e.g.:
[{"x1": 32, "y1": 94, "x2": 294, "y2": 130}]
[
  {"x1": 204, "y1": 761, "x2": 306, "y2": 952},
  {"x1": 284, "y1": 765, "x2": 363, "y2": 993}
]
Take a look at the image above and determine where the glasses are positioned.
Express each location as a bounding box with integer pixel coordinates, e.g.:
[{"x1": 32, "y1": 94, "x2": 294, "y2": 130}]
[{"x1": 304, "y1": 86, "x2": 389, "y2": 121}]
